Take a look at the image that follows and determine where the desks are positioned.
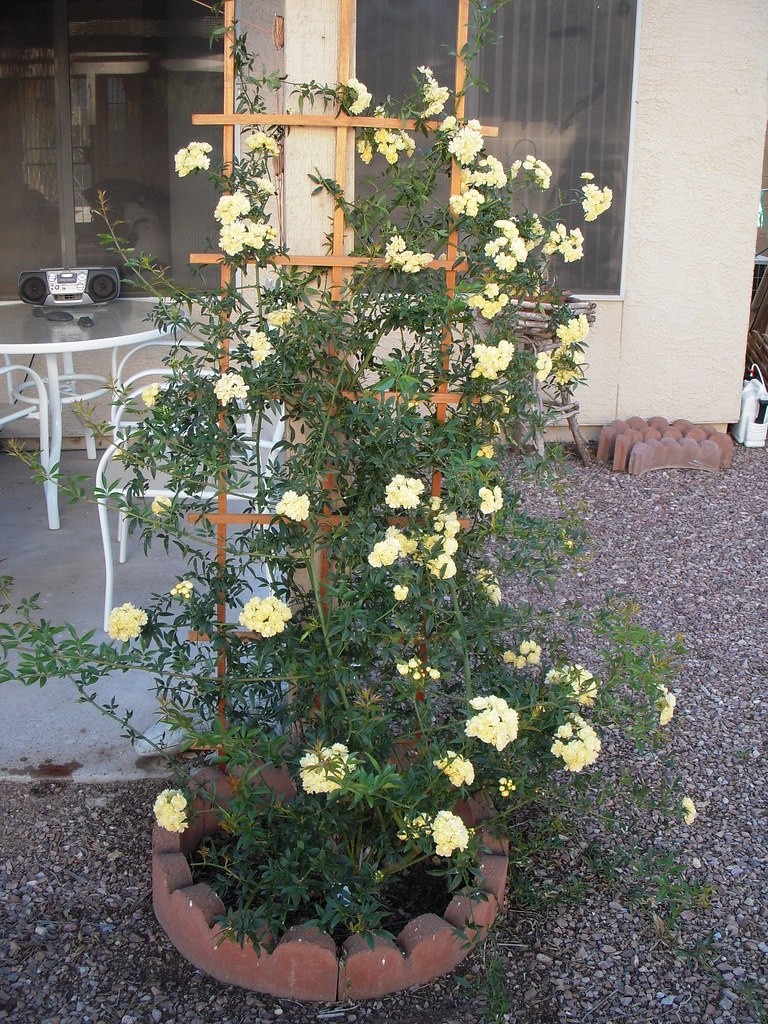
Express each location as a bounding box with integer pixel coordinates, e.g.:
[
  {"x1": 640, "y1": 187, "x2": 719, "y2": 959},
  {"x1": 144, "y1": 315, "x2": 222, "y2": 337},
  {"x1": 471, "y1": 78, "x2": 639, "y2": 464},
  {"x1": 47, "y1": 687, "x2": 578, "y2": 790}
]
[{"x1": 1, "y1": 293, "x2": 189, "y2": 535}]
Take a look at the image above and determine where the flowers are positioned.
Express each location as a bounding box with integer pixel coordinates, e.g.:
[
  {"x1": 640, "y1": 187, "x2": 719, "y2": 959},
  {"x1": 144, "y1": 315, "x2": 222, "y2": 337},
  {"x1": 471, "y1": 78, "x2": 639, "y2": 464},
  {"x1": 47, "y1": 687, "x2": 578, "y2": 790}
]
[{"x1": 1, "y1": 0, "x2": 713, "y2": 957}]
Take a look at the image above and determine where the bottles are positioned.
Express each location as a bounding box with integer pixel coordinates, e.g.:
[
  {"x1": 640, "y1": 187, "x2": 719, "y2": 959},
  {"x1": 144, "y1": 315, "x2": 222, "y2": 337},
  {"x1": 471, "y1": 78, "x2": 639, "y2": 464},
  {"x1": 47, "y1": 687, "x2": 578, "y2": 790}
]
[{"x1": 732, "y1": 363, "x2": 767, "y2": 448}]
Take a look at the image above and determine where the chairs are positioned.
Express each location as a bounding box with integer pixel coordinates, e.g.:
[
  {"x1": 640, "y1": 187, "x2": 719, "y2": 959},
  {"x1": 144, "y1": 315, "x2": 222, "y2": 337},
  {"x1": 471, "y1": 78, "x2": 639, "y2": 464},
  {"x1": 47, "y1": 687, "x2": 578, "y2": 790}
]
[{"x1": 92, "y1": 324, "x2": 301, "y2": 635}]
